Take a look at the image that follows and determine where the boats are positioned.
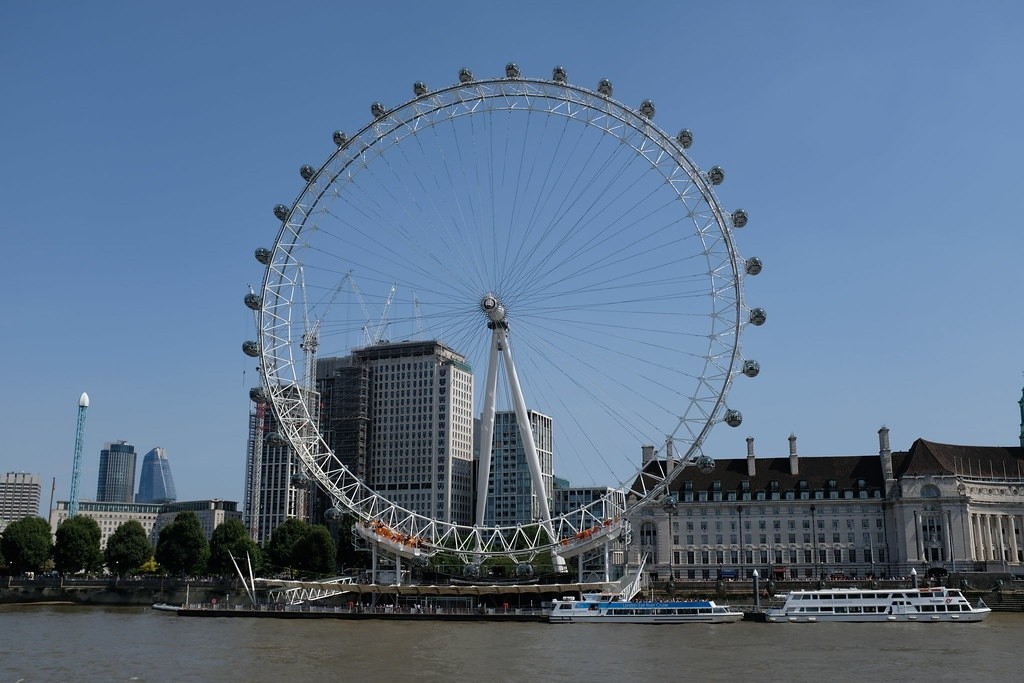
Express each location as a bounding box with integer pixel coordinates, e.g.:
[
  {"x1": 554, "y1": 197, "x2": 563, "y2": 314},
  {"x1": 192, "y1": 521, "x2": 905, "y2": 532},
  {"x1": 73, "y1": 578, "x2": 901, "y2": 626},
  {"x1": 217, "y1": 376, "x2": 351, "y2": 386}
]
[
  {"x1": 763, "y1": 587, "x2": 992, "y2": 623},
  {"x1": 548, "y1": 595, "x2": 745, "y2": 624},
  {"x1": 152, "y1": 603, "x2": 186, "y2": 611}
]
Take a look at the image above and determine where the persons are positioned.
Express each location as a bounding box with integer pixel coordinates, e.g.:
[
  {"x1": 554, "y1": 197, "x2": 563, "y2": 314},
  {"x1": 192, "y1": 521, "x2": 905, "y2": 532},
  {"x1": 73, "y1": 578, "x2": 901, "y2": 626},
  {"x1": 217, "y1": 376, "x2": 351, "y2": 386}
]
[
  {"x1": 630, "y1": 598, "x2": 709, "y2": 613},
  {"x1": 364, "y1": 519, "x2": 434, "y2": 552}
]
